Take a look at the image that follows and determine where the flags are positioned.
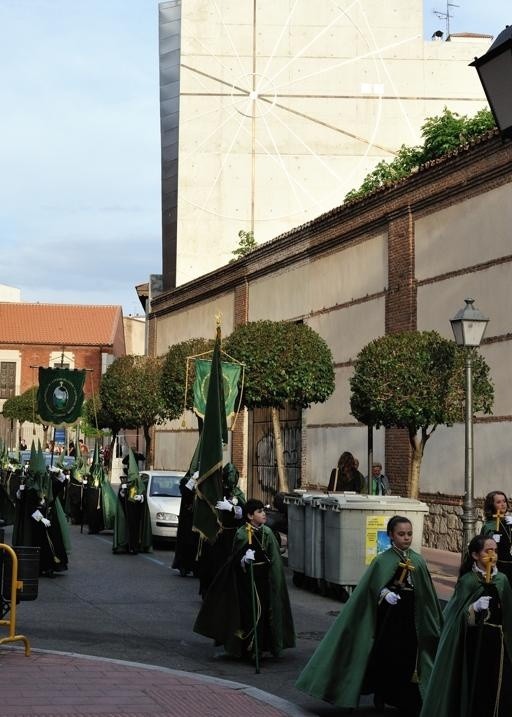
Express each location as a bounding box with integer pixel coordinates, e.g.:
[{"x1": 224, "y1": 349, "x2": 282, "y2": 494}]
[{"x1": 191, "y1": 325, "x2": 228, "y2": 562}]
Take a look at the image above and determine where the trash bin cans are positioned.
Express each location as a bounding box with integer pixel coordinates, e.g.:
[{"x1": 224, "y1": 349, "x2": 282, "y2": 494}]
[
  {"x1": 2, "y1": 546, "x2": 41, "y2": 601},
  {"x1": 282, "y1": 489, "x2": 430, "y2": 603}
]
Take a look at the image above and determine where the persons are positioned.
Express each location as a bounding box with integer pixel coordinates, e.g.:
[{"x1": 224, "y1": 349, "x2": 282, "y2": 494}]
[
  {"x1": 192, "y1": 498, "x2": 296, "y2": 668},
  {"x1": 363, "y1": 463, "x2": 392, "y2": 496},
  {"x1": 0, "y1": 437, "x2": 155, "y2": 579},
  {"x1": 172, "y1": 462, "x2": 246, "y2": 601},
  {"x1": 293, "y1": 515, "x2": 446, "y2": 715},
  {"x1": 326, "y1": 452, "x2": 364, "y2": 494},
  {"x1": 480, "y1": 491, "x2": 511, "y2": 582},
  {"x1": 421, "y1": 535, "x2": 511, "y2": 717}
]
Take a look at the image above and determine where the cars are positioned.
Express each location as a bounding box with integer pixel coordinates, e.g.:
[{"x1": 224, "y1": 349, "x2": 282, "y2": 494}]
[
  {"x1": 137, "y1": 470, "x2": 189, "y2": 544},
  {"x1": 18, "y1": 451, "x2": 75, "y2": 488}
]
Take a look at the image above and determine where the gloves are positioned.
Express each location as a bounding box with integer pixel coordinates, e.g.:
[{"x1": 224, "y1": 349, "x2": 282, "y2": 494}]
[
  {"x1": 134, "y1": 494, "x2": 142, "y2": 500},
  {"x1": 492, "y1": 534, "x2": 502, "y2": 542},
  {"x1": 504, "y1": 515, "x2": 512, "y2": 524},
  {"x1": 41, "y1": 517, "x2": 51, "y2": 527},
  {"x1": 384, "y1": 592, "x2": 401, "y2": 605},
  {"x1": 215, "y1": 496, "x2": 233, "y2": 512},
  {"x1": 243, "y1": 548, "x2": 256, "y2": 561},
  {"x1": 473, "y1": 596, "x2": 492, "y2": 612},
  {"x1": 192, "y1": 471, "x2": 199, "y2": 479}
]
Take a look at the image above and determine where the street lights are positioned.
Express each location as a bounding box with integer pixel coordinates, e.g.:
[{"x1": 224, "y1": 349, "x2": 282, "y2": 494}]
[{"x1": 449, "y1": 296, "x2": 490, "y2": 563}]
